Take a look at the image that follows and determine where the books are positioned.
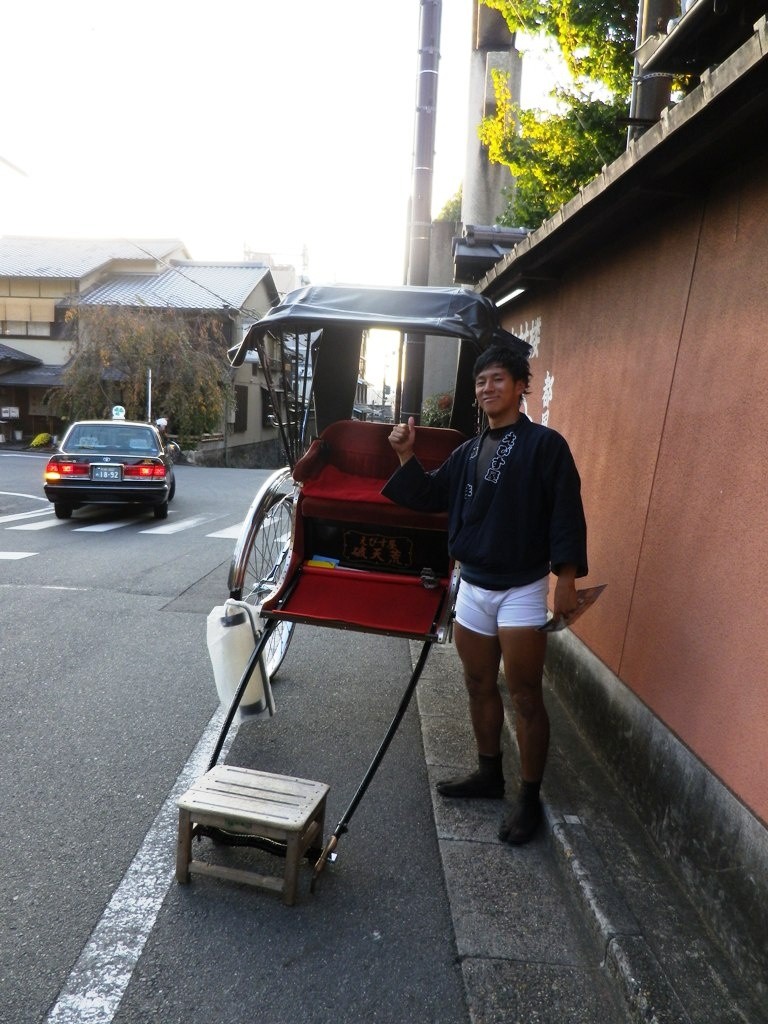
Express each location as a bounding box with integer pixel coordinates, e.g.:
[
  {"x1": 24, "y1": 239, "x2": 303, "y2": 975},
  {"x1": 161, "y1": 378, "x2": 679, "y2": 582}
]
[{"x1": 537, "y1": 583, "x2": 605, "y2": 634}]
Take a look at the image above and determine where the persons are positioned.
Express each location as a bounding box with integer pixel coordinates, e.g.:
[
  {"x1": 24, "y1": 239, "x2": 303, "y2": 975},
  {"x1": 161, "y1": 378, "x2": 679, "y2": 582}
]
[{"x1": 379, "y1": 343, "x2": 590, "y2": 846}]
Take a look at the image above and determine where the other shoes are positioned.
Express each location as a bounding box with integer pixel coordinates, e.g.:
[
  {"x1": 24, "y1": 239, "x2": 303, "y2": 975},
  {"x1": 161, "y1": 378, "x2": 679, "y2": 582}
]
[
  {"x1": 435, "y1": 770, "x2": 507, "y2": 797},
  {"x1": 498, "y1": 791, "x2": 542, "y2": 844}
]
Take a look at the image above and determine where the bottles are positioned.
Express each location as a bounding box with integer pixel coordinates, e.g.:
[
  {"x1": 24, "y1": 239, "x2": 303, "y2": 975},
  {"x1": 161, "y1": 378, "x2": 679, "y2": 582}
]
[{"x1": 220, "y1": 608, "x2": 265, "y2": 717}]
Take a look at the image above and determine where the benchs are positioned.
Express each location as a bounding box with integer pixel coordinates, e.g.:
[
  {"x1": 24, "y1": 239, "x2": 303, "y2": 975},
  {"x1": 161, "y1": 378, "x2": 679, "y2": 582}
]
[{"x1": 290, "y1": 417, "x2": 465, "y2": 561}]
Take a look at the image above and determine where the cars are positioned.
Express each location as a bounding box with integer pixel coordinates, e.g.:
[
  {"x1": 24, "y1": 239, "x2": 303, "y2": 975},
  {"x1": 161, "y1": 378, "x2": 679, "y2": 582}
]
[{"x1": 43, "y1": 404, "x2": 181, "y2": 520}]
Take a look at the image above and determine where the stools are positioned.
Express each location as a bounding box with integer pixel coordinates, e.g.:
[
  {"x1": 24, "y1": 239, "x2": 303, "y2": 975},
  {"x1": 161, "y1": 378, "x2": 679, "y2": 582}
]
[{"x1": 174, "y1": 765, "x2": 329, "y2": 907}]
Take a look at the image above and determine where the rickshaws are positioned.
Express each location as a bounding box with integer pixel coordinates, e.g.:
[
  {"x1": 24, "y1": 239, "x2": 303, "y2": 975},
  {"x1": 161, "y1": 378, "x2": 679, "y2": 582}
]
[{"x1": 186, "y1": 277, "x2": 501, "y2": 898}]
